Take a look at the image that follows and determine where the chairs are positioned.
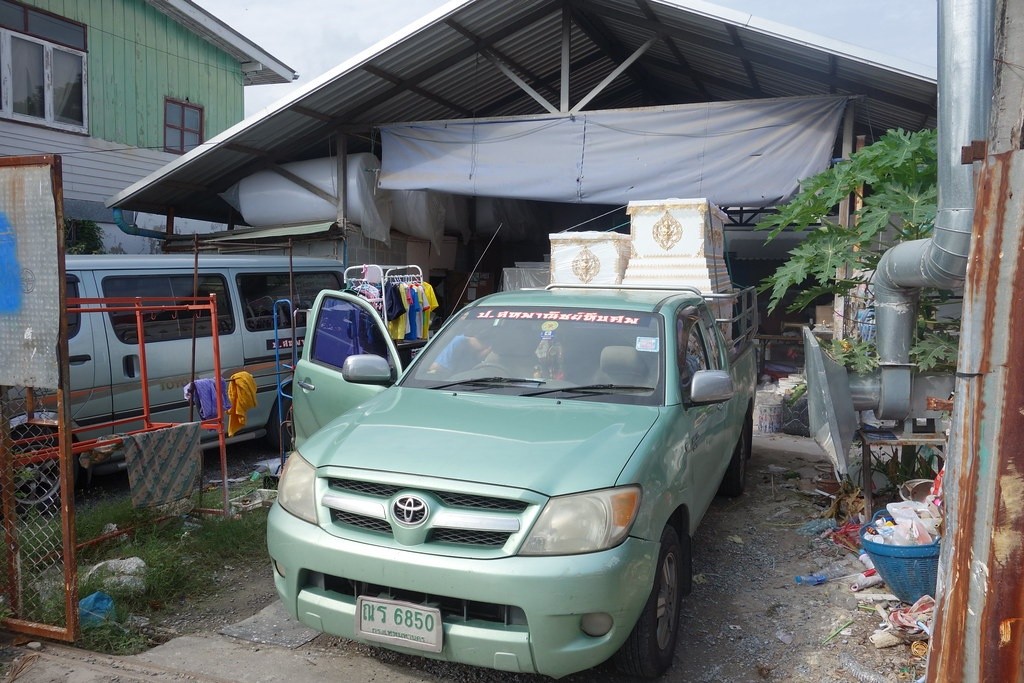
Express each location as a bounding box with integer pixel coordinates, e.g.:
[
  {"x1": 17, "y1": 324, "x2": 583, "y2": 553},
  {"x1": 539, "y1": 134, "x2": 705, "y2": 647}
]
[{"x1": 590, "y1": 345, "x2": 649, "y2": 390}]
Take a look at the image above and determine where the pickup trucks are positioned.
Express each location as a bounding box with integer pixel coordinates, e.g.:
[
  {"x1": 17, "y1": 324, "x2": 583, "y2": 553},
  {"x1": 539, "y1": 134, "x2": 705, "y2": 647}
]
[{"x1": 261, "y1": 283, "x2": 763, "y2": 683}]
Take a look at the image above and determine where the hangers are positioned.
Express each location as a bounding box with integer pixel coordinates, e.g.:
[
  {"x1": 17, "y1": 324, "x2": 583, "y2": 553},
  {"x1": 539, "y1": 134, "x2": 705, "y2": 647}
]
[
  {"x1": 388, "y1": 274, "x2": 423, "y2": 287},
  {"x1": 350, "y1": 278, "x2": 372, "y2": 287}
]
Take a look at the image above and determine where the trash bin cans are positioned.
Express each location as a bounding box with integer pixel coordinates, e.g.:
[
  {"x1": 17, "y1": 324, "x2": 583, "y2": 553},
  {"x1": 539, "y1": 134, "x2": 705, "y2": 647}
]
[{"x1": 859, "y1": 509, "x2": 942, "y2": 605}]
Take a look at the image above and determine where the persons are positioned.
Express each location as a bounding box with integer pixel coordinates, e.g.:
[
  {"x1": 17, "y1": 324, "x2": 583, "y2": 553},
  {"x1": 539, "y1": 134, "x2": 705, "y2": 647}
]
[{"x1": 429, "y1": 329, "x2": 496, "y2": 373}]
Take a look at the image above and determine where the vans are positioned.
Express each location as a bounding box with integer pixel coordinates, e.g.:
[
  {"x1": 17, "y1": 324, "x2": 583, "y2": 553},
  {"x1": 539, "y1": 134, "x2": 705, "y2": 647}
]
[{"x1": 0, "y1": 254, "x2": 354, "y2": 518}]
